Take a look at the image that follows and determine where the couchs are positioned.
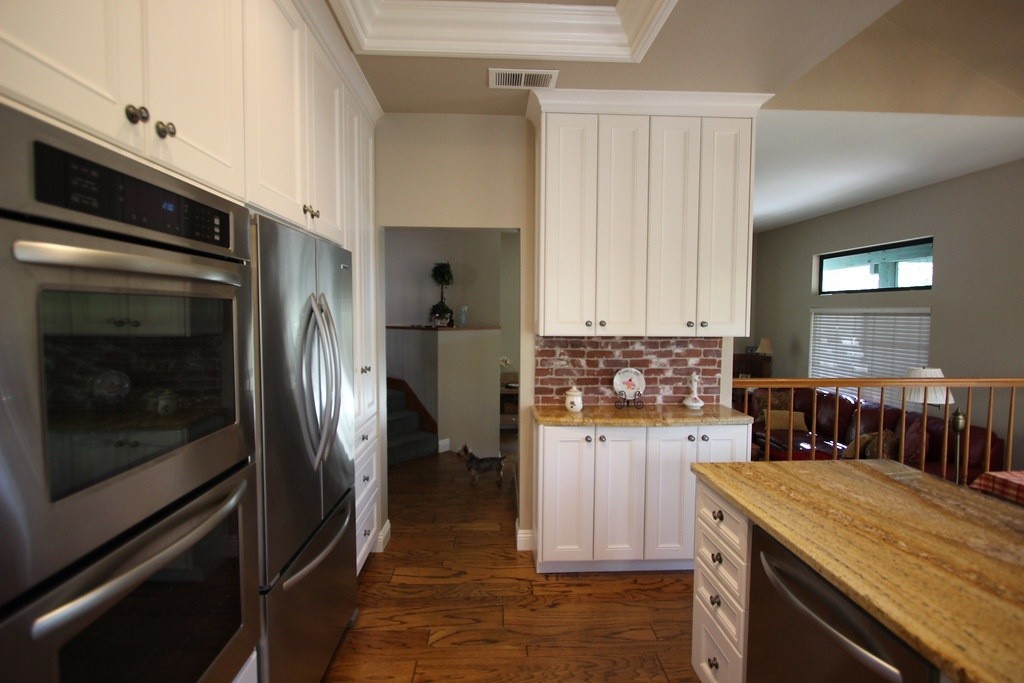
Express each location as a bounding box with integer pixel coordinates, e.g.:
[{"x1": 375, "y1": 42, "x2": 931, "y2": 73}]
[{"x1": 750, "y1": 386, "x2": 1005, "y2": 487}]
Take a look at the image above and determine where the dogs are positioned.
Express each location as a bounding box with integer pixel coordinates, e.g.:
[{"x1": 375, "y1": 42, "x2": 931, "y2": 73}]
[{"x1": 457, "y1": 443, "x2": 507, "y2": 487}]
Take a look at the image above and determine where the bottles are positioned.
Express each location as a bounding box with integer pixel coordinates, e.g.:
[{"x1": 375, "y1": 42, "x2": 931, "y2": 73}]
[{"x1": 682, "y1": 372, "x2": 704, "y2": 409}]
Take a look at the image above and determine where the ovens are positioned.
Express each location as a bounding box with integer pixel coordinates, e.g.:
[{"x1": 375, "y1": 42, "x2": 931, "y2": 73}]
[{"x1": 0, "y1": 100, "x2": 254, "y2": 683}]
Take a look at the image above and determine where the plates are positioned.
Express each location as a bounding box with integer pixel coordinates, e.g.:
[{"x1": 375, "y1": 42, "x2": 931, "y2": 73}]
[{"x1": 612, "y1": 367, "x2": 646, "y2": 400}]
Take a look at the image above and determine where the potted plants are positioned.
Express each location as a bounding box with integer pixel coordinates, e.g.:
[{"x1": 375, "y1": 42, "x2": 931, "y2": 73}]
[{"x1": 429, "y1": 262, "x2": 454, "y2": 327}]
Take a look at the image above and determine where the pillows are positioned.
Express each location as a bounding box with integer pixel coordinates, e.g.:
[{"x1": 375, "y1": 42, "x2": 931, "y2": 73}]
[
  {"x1": 753, "y1": 388, "x2": 795, "y2": 424},
  {"x1": 863, "y1": 429, "x2": 899, "y2": 460},
  {"x1": 762, "y1": 408, "x2": 808, "y2": 432},
  {"x1": 842, "y1": 432, "x2": 879, "y2": 458}
]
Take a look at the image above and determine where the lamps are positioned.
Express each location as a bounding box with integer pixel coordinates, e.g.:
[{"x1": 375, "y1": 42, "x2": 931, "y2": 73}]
[
  {"x1": 756, "y1": 338, "x2": 775, "y2": 358},
  {"x1": 898, "y1": 366, "x2": 955, "y2": 410}
]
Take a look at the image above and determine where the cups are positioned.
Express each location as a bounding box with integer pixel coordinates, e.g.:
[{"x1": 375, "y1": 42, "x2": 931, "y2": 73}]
[
  {"x1": 457, "y1": 303, "x2": 470, "y2": 328},
  {"x1": 565, "y1": 386, "x2": 583, "y2": 413}
]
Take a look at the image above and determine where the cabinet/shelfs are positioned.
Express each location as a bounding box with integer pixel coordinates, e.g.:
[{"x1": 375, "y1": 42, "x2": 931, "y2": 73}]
[
  {"x1": 336, "y1": 77, "x2": 380, "y2": 577},
  {"x1": 646, "y1": 424, "x2": 752, "y2": 571},
  {"x1": 533, "y1": 113, "x2": 757, "y2": 337},
  {"x1": 500, "y1": 372, "x2": 519, "y2": 434},
  {"x1": 0, "y1": 0, "x2": 247, "y2": 204},
  {"x1": 691, "y1": 458, "x2": 750, "y2": 683},
  {"x1": 531, "y1": 427, "x2": 646, "y2": 573},
  {"x1": 242, "y1": 0, "x2": 345, "y2": 245}
]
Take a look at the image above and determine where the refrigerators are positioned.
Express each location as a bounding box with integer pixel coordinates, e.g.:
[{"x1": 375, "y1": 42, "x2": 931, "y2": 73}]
[{"x1": 256, "y1": 210, "x2": 358, "y2": 683}]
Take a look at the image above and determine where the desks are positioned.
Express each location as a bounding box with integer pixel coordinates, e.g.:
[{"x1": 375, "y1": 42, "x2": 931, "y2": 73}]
[{"x1": 970, "y1": 470, "x2": 1024, "y2": 508}]
